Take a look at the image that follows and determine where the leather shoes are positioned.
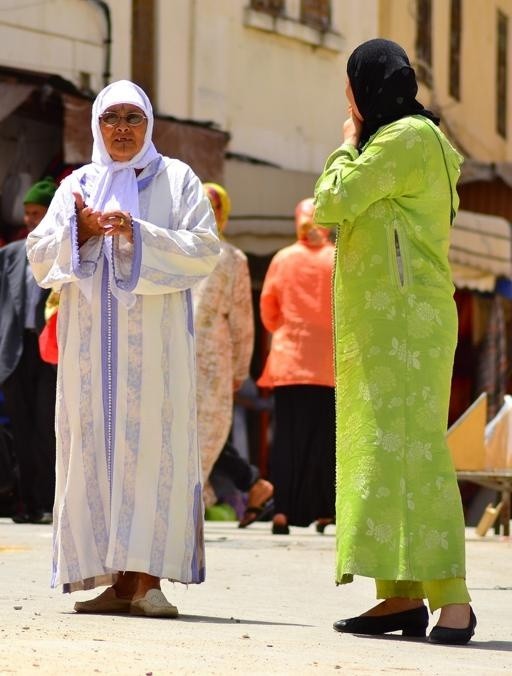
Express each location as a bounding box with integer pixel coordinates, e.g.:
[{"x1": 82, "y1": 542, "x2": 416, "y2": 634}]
[
  {"x1": 75, "y1": 587, "x2": 132, "y2": 612},
  {"x1": 129, "y1": 589, "x2": 179, "y2": 618}
]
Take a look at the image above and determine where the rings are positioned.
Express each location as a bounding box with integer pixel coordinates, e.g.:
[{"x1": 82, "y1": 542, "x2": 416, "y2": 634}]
[{"x1": 119, "y1": 217, "x2": 125, "y2": 225}]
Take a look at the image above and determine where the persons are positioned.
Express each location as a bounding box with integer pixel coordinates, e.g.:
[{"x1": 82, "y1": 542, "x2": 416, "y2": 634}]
[
  {"x1": 254, "y1": 196, "x2": 336, "y2": 537},
  {"x1": 188, "y1": 181, "x2": 255, "y2": 486},
  {"x1": 0, "y1": 178, "x2": 58, "y2": 525},
  {"x1": 312, "y1": 36, "x2": 479, "y2": 645},
  {"x1": 24, "y1": 79, "x2": 222, "y2": 617},
  {"x1": 218, "y1": 441, "x2": 274, "y2": 530}
]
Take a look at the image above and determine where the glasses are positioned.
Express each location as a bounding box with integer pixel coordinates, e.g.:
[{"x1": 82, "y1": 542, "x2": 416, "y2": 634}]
[{"x1": 98, "y1": 111, "x2": 148, "y2": 127}]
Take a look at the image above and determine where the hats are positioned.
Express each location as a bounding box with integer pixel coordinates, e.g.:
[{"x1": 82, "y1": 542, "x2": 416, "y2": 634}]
[{"x1": 23, "y1": 177, "x2": 57, "y2": 206}]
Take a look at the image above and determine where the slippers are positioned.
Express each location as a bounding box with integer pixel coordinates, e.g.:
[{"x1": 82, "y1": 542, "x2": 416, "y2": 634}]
[{"x1": 238, "y1": 499, "x2": 275, "y2": 528}]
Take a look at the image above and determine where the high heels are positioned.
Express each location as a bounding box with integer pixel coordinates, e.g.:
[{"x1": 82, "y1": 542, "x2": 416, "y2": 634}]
[
  {"x1": 428, "y1": 604, "x2": 476, "y2": 644},
  {"x1": 333, "y1": 605, "x2": 429, "y2": 637}
]
